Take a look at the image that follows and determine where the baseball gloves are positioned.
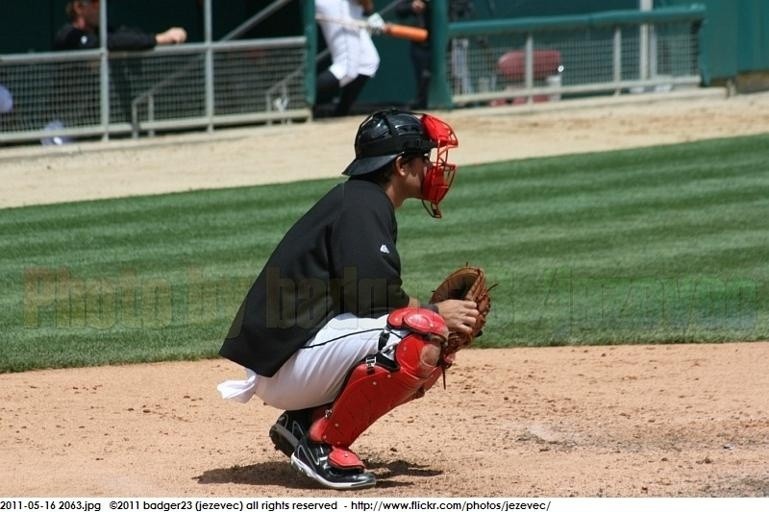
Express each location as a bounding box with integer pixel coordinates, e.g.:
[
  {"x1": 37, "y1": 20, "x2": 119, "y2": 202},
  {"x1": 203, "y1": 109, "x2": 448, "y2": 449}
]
[{"x1": 429, "y1": 267, "x2": 492, "y2": 354}]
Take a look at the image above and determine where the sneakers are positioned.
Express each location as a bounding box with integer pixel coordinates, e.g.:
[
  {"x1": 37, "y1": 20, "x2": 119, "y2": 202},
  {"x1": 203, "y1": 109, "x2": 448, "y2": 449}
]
[
  {"x1": 290, "y1": 433, "x2": 377, "y2": 489},
  {"x1": 269, "y1": 410, "x2": 313, "y2": 458},
  {"x1": 274, "y1": 95, "x2": 292, "y2": 123}
]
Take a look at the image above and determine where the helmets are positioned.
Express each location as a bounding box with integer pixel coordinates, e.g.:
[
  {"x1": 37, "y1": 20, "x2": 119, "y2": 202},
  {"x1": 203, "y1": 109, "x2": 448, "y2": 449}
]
[{"x1": 341, "y1": 107, "x2": 459, "y2": 219}]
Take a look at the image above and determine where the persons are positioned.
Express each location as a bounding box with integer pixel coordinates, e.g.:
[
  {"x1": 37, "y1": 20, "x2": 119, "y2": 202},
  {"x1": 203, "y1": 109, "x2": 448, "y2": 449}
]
[
  {"x1": 274, "y1": 0, "x2": 388, "y2": 120},
  {"x1": 51, "y1": 0, "x2": 190, "y2": 125},
  {"x1": 218, "y1": 103, "x2": 481, "y2": 493},
  {"x1": 395, "y1": 1, "x2": 472, "y2": 112}
]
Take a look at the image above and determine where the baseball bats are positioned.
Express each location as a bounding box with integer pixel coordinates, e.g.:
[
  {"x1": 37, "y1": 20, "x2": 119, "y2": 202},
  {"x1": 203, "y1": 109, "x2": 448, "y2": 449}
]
[{"x1": 314, "y1": 13, "x2": 427, "y2": 41}]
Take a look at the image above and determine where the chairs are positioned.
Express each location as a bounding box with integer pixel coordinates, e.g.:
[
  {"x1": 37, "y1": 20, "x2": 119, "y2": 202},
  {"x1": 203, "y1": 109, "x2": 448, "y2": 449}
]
[{"x1": 488, "y1": 50, "x2": 564, "y2": 106}]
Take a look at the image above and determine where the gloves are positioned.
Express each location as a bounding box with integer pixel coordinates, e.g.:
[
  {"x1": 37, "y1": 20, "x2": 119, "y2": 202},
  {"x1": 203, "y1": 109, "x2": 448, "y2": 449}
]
[{"x1": 368, "y1": 12, "x2": 385, "y2": 36}]
